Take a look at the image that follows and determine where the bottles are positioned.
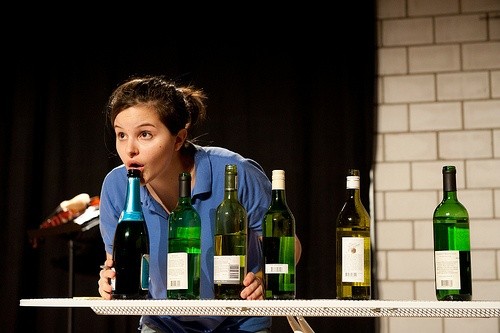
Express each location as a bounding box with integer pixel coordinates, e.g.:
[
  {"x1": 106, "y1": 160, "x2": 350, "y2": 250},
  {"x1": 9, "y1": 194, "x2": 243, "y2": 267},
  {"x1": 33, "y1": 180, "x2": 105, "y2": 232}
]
[
  {"x1": 214, "y1": 165, "x2": 248, "y2": 300},
  {"x1": 107, "y1": 168, "x2": 150, "y2": 300},
  {"x1": 262, "y1": 170, "x2": 296, "y2": 300},
  {"x1": 433, "y1": 166, "x2": 473, "y2": 301},
  {"x1": 336, "y1": 169, "x2": 372, "y2": 300},
  {"x1": 166, "y1": 173, "x2": 202, "y2": 299}
]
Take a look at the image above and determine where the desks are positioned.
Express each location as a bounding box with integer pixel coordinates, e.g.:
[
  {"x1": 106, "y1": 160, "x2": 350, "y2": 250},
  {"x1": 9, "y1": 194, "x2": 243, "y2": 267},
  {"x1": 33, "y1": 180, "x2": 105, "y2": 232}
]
[{"x1": 19, "y1": 297, "x2": 500, "y2": 333}]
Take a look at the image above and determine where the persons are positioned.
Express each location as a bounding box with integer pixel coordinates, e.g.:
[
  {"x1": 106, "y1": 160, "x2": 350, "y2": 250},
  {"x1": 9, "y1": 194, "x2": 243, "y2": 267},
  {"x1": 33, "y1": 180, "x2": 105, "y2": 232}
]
[{"x1": 98, "y1": 77, "x2": 302, "y2": 333}]
